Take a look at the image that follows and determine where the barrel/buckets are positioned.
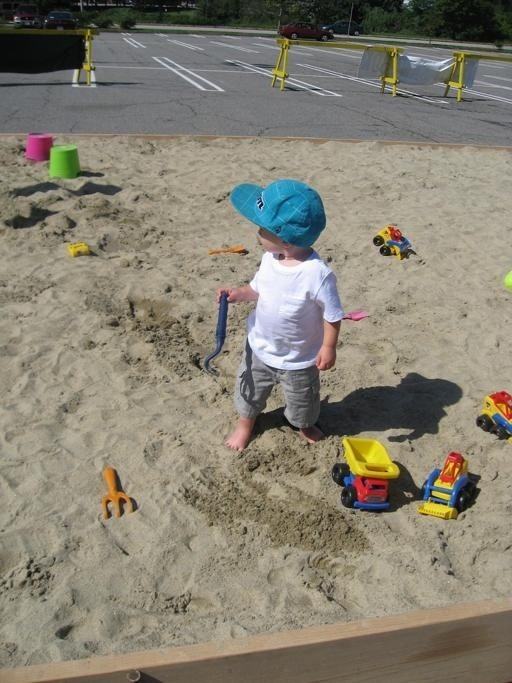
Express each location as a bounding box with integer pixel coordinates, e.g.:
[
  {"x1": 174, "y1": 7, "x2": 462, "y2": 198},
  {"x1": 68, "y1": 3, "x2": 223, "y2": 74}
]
[
  {"x1": 24, "y1": 131, "x2": 54, "y2": 161},
  {"x1": 49, "y1": 144, "x2": 81, "y2": 179}
]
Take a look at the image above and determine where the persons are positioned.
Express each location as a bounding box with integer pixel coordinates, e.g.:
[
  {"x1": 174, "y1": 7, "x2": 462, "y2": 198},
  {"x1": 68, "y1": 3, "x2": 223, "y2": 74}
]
[{"x1": 213, "y1": 179, "x2": 343, "y2": 452}]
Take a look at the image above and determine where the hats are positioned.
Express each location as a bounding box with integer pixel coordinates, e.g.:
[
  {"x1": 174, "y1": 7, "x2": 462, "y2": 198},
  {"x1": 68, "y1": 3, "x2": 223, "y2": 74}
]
[{"x1": 229, "y1": 178, "x2": 327, "y2": 248}]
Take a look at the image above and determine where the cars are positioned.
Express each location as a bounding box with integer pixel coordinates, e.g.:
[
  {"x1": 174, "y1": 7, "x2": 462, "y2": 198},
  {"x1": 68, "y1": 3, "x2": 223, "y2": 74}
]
[
  {"x1": 13, "y1": 4, "x2": 79, "y2": 30},
  {"x1": 279, "y1": 20, "x2": 365, "y2": 40}
]
